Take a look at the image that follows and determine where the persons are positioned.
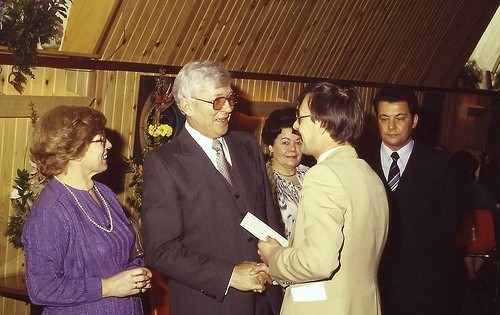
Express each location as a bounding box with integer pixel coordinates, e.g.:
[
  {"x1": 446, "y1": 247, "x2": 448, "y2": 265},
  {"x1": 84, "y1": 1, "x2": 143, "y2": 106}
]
[
  {"x1": 454, "y1": 148, "x2": 500, "y2": 291},
  {"x1": 21, "y1": 105, "x2": 153, "y2": 315},
  {"x1": 262, "y1": 107, "x2": 311, "y2": 242},
  {"x1": 257, "y1": 82, "x2": 390, "y2": 315},
  {"x1": 358, "y1": 83, "x2": 459, "y2": 315},
  {"x1": 141, "y1": 62, "x2": 287, "y2": 315}
]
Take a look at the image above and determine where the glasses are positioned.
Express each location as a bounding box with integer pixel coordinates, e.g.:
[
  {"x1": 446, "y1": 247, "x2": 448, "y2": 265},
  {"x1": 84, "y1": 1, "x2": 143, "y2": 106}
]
[
  {"x1": 296, "y1": 111, "x2": 312, "y2": 125},
  {"x1": 88, "y1": 135, "x2": 107, "y2": 147},
  {"x1": 190, "y1": 89, "x2": 239, "y2": 110}
]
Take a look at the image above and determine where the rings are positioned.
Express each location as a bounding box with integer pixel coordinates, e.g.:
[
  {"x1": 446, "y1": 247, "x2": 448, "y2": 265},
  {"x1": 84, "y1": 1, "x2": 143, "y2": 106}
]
[
  {"x1": 259, "y1": 282, "x2": 262, "y2": 285},
  {"x1": 136, "y1": 282, "x2": 137, "y2": 289}
]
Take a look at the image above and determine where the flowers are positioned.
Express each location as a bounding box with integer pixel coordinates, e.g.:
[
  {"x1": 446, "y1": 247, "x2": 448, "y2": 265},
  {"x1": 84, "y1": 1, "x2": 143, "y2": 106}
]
[{"x1": 140, "y1": 75, "x2": 175, "y2": 140}]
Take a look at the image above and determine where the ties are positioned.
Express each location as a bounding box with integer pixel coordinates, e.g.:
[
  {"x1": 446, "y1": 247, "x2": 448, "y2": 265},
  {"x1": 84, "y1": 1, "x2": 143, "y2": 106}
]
[
  {"x1": 211, "y1": 138, "x2": 234, "y2": 186},
  {"x1": 388, "y1": 151, "x2": 402, "y2": 191}
]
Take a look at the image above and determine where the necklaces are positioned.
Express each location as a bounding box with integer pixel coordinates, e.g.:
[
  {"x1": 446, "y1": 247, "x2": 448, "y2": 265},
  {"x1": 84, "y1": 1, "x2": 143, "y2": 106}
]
[
  {"x1": 278, "y1": 171, "x2": 295, "y2": 177},
  {"x1": 54, "y1": 176, "x2": 113, "y2": 233}
]
